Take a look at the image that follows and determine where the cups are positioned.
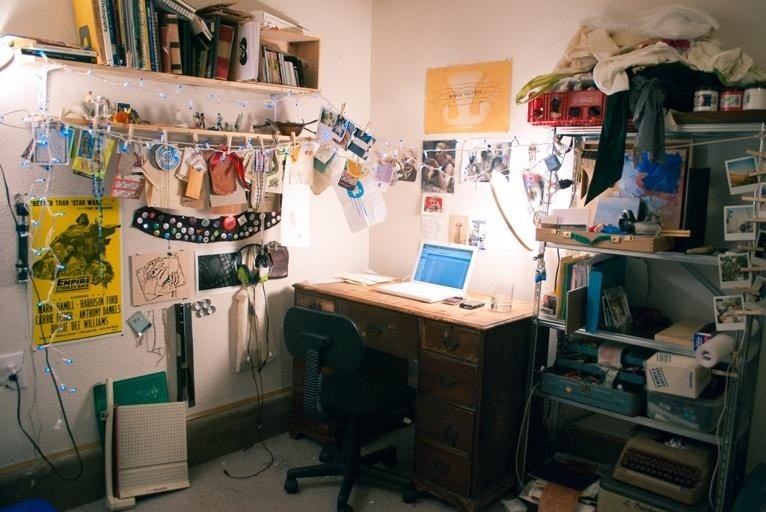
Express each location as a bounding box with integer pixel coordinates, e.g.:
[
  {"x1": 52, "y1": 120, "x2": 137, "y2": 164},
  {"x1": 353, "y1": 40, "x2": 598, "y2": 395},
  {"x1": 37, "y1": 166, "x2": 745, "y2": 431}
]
[{"x1": 489, "y1": 283, "x2": 514, "y2": 312}]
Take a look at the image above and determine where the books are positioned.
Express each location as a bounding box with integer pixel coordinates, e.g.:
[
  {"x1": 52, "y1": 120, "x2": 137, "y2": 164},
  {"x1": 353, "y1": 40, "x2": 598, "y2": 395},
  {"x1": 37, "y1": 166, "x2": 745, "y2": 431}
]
[
  {"x1": 261, "y1": 45, "x2": 304, "y2": 85},
  {"x1": 0, "y1": 1, "x2": 260, "y2": 83}
]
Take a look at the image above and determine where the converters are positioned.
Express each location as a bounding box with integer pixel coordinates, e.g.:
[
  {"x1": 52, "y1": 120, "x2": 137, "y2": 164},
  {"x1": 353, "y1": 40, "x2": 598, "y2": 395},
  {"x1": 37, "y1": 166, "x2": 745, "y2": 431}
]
[{"x1": 545, "y1": 155, "x2": 562, "y2": 171}]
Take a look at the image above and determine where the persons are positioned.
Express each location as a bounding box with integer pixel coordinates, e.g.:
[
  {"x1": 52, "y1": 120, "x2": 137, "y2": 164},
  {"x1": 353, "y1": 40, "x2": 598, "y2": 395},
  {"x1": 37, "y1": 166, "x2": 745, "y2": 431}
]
[
  {"x1": 34, "y1": 214, "x2": 114, "y2": 285},
  {"x1": 426, "y1": 142, "x2": 453, "y2": 191}
]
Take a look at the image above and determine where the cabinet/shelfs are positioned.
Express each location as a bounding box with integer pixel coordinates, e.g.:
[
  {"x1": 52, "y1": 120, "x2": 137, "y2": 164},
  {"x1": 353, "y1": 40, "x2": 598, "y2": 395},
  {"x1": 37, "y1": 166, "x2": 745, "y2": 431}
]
[
  {"x1": 16, "y1": 27, "x2": 322, "y2": 143},
  {"x1": 530, "y1": 123, "x2": 766, "y2": 512}
]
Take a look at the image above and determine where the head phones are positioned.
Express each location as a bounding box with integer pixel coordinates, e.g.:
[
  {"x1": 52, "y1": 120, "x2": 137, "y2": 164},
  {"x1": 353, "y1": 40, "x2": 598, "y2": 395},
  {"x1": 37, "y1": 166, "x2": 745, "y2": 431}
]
[{"x1": 232, "y1": 243, "x2": 274, "y2": 287}]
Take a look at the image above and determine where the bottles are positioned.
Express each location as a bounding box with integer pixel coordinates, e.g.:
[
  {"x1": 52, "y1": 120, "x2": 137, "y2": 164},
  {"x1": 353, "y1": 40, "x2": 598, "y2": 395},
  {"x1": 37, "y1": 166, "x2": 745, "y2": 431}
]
[
  {"x1": 693, "y1": 87, "x2": 719, "y2": 112},
  {"x1": 743, "y1": 83, "x2": 766, "y2": 110},
  {"x1": 720, "y1": 86, "x2": 743, "y2": 112}
]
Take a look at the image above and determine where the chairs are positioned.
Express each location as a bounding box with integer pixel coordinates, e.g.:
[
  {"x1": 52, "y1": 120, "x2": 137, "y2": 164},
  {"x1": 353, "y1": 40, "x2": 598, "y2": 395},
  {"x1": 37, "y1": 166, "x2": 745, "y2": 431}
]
[{"x1": 284, "y1": 306, "x2": 418, "y2": 512}]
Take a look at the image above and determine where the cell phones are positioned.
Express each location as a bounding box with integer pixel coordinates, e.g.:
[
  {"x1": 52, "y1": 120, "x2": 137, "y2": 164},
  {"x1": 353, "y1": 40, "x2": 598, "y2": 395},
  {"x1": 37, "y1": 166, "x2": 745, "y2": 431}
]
[{"x1": 460, "y1": 300, "x2": 484, "y2": 310}]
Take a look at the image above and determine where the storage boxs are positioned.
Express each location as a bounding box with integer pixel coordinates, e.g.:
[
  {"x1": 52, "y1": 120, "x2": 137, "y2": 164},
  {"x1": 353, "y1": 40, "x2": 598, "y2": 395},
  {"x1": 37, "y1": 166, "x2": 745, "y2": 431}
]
[
  {"x1": 647, "y1": 351, "x2": 712, "y2": 400},
  {"x1": 644, "y1": 382, "x2": 730, "y2": 433}
]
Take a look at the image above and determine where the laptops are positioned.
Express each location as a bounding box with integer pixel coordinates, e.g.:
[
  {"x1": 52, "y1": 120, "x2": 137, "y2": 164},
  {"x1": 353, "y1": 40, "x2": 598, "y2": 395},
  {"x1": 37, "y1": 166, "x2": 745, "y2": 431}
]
[{"x1": 371, "y1": 238, "x2": 479, "y2": 303}]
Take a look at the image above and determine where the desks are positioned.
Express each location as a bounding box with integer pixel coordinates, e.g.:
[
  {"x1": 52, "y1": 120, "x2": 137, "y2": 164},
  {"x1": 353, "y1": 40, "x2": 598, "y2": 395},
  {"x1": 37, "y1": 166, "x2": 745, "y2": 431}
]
[{"x1": 289, "y1": 276, "x2": 550, "y2": 512}]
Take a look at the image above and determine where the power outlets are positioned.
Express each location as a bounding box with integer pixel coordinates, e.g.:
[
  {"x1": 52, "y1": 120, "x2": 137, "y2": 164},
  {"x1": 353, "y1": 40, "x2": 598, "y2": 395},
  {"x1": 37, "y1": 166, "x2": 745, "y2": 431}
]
[{"x1": 0, "y1": 351, "x2": 28, "y2": 390}]
